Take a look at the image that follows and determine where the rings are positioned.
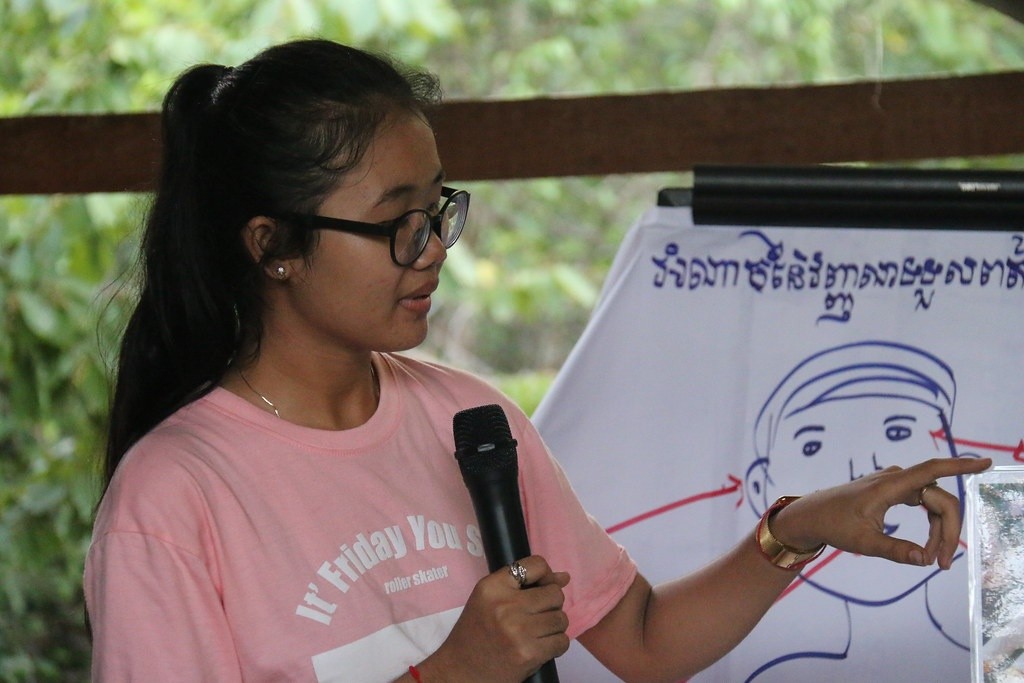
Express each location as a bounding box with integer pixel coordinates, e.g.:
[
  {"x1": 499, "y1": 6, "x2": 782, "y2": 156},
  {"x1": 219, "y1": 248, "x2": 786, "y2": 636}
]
[
  {"x1": 510, "y1": 562, "x2": 526, "y2": 585},
  {"x1": 918, "y1": 483, "x2": 935, "y2": 503}
]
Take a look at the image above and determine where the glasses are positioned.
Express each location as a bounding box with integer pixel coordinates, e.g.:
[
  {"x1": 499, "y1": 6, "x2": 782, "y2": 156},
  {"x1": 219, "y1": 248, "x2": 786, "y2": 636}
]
[{"x1": 246, "y1": 184, "x2": 471, "y2": 267}]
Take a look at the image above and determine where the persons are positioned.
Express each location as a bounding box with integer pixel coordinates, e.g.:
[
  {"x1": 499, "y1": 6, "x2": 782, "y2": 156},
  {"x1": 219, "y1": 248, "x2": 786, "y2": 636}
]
[{"x1": 82, "y1": 39, "x2": 991, "y2": 683}]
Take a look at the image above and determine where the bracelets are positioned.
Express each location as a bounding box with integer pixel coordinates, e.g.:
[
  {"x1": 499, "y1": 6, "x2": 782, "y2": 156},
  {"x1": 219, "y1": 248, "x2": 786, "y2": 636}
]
[
  {"x1": 409, "y1": 666, "x2": 422, "y2": 683},
  {"x1": 755, "y1": 495, "x2": 827, "y2": 569}
]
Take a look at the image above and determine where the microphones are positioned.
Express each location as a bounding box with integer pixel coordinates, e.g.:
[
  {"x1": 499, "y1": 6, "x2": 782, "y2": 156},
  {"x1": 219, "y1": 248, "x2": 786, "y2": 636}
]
[{"x1": 454, "y1": 404, "x2": 560, "y2": 683}]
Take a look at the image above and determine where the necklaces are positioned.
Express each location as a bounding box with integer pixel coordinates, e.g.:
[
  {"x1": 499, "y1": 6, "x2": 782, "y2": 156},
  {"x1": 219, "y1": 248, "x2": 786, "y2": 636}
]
[{"x1": 238, "y1": 368, "x2": 280, "y2": 419}]
